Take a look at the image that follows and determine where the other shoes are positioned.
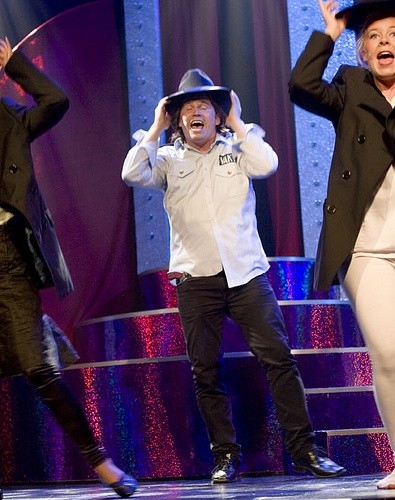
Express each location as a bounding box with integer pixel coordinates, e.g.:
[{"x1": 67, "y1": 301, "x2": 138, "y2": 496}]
[{"x1": 108, "y1": 474, "x2": 139, "y2": 497}]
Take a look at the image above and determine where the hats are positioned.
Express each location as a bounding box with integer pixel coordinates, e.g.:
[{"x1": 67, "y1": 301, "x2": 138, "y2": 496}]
[
  {"x1": 334, "y1": 0, "x2": 395, "y2": 29},
  {"x1": 165, "y1": 68, "x2": 231, "y2": 114}
]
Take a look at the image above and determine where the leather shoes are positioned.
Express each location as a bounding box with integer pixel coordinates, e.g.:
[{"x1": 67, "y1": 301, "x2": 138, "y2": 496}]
[
  {"x1": 211, "y1": 458, "x2": 239, "y2": 482},
  {"x1": 292, "y1": 443, "x2": 348, "y2": 478}
]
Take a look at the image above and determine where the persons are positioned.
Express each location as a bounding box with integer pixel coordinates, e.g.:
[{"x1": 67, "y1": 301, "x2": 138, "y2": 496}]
[
  {"x1": 0, "y1": 37, "x2": 138, "y2": 498},
  {"x1": 286, "y1": 0, "x2": 395, "y2": 491},
  {"x1": 121, "y1": 67, "x2": 346, "y2": 484}
]
